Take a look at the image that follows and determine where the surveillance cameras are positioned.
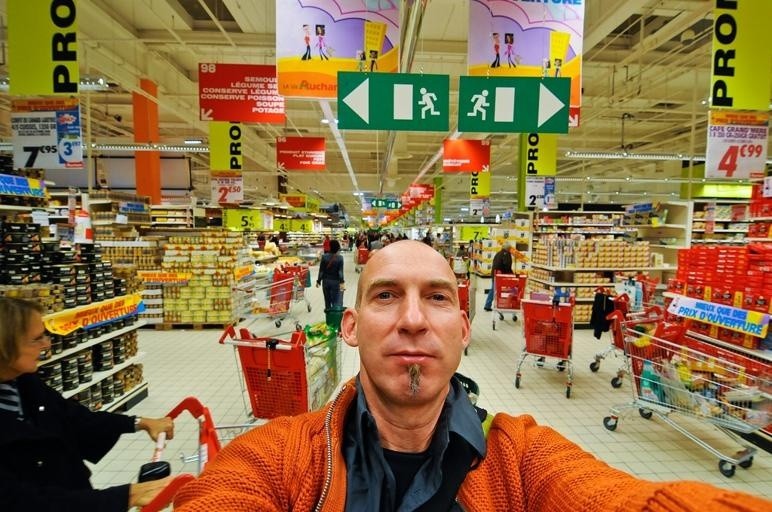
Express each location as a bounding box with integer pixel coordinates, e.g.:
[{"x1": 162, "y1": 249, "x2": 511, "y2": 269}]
[{"x1": 681, "y1": 29, "x2": 696, "y2": 45}]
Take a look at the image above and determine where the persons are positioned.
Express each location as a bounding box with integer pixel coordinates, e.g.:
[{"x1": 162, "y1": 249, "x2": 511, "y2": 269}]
[
  {"x1": 173, "y1": 238, "x2": 771, "y2": 512},
  {"x1": 1, "y1": 296, "x2": 183, "y2": 512},
  {"x1": 256, "y1": 225, "x2": 515, "y2": 338}
]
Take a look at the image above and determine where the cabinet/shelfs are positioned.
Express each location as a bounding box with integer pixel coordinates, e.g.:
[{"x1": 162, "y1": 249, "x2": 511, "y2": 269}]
[
  {"x1": 691, "y1": 200, "x2": 750, "y2": 246},
  {"x1": 1, "y1": 200, "x2": 164, "y2": 412},
  {"x1": 529, "y1": 198, "x2": 696, "y2": 330},
  {"x1": 747, "y1": 216, "x2": 771, "y2": 242}
]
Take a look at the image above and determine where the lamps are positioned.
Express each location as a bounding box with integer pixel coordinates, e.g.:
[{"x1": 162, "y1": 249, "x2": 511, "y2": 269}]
[
  {"x1": 0, "y1": 40, "x2": 111, "y2": 94},
  {"x1": 82, "y1": 142, "x2": 211, "y2": 153},
  {"x1": 507, "y1": 147, "x2": 747, "y2": 197}
]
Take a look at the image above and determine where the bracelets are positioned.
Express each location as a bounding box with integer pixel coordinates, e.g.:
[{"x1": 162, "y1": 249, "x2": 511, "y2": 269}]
[{"x1": 135, "y1": 417, "x2": 141, "y2": 433}]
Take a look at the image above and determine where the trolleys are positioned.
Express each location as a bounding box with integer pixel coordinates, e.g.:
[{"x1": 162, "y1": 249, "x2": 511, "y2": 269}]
[
  {"x1": 231, "y1": 256, "x2": 312, "y2": 331},
  {"x1": 353, "y1": 248, "x2": 369, "y2": 273},
  {"x1": 492, "y1": 268, "x2": 527, "y2": 331},
  {"x1": 590, "y1": 269, "x2": 772, "y2": 478},
  {"x1": 135, "y1": 394, "x2": 265, "y2": 512},
  {"x1": 514, "y1": 287, "x2": 578, "y2": 400},
  {"x1": 449, "y1": 254, "x2": 477, "y2": 357},
  {"x1": 220, "y1": 319, "x2": 342, "y2": 423}
]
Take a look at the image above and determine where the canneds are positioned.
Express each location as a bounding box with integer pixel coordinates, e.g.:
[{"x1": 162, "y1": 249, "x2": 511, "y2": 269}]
[{"x1": 0, "y1": 151, "x2": 143, "y2": 412}]
